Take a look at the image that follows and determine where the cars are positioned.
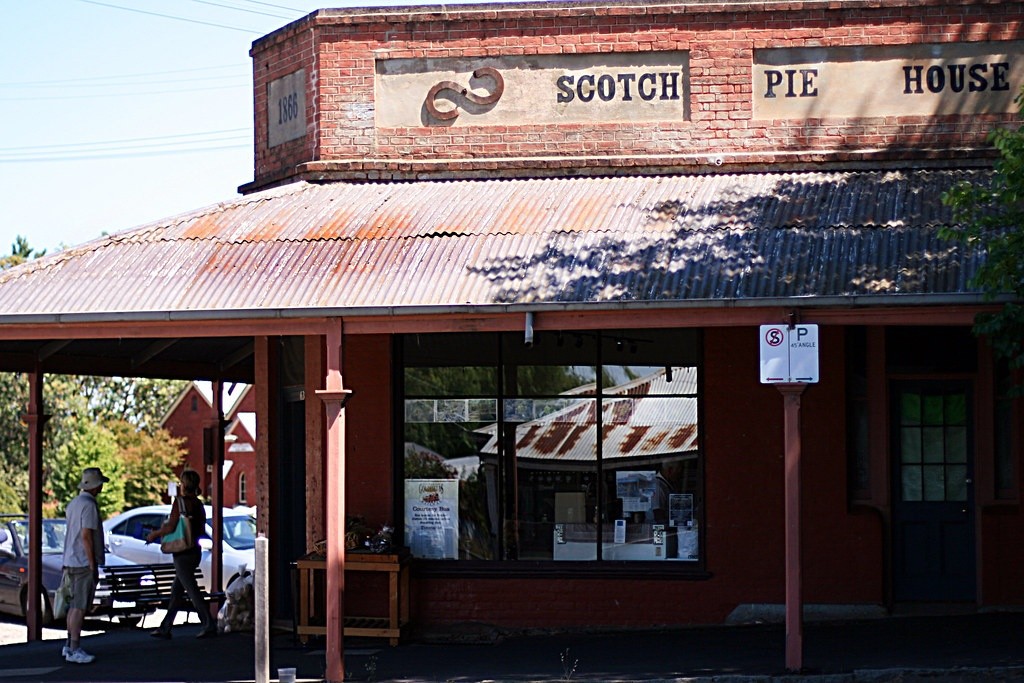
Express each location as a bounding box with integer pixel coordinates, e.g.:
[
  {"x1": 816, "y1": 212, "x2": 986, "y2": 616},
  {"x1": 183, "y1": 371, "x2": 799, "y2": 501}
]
[
  {"x1": 103, "y1": 505, "x2": 256, "y2": 597},
  {"x1": 1, "y1": 512, "x2": 160, "y2": 624}
]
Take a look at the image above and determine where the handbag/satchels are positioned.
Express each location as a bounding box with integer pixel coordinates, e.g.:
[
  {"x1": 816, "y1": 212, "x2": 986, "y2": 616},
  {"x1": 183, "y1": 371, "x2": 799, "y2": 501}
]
[
  {"x1": 53, "y1": 569, "x2": 72, "y2": 619},
  {"x1": 161, "y1": 496, "x2": 191, "y2": 554}
]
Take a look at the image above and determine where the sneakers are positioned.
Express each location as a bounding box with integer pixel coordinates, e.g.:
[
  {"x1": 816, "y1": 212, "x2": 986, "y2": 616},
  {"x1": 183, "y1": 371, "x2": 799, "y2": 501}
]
[
  {"x1": 66, "y1": 648, "x2": 95, "y2": 663},
  {"x1": 62, "y1": 646, "x2": 69, "y2": 656}
]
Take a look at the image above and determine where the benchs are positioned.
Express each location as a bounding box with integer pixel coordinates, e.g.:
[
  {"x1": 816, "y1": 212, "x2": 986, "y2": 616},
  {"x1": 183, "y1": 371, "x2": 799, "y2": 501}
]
[{"x1": 100, "y1": 560, "x2": 226, "y2": 631}]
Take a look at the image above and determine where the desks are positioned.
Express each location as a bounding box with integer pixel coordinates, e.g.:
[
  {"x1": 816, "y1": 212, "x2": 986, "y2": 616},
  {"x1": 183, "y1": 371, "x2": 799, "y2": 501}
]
[{"x1": 296, "y1": 544, "x2": 414, "y2": 649}]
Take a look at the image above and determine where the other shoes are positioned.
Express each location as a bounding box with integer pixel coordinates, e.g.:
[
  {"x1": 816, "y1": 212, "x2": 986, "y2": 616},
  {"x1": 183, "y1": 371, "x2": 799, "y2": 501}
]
[
  {"x1": 150, "y1": 631, "x2": 172, "y2": 640},
  {"x1": 196, "y1": 631, "x2": 217, "y2": 638}
]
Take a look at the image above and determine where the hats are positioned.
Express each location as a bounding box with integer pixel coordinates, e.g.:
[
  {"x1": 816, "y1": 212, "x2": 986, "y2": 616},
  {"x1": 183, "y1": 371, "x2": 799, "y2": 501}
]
[{"x1": 77, "y1": 468, "x2": 109, "y2": 490}]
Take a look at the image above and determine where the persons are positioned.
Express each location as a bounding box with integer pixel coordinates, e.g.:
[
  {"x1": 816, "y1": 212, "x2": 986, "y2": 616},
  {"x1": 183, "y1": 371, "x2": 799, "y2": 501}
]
[
  {"x1": 60, "y1": 466, "x2": 111, "y2": 664},
  {"x1": 145, "y1": 470, "x2": 220, "y2": 639}
]
